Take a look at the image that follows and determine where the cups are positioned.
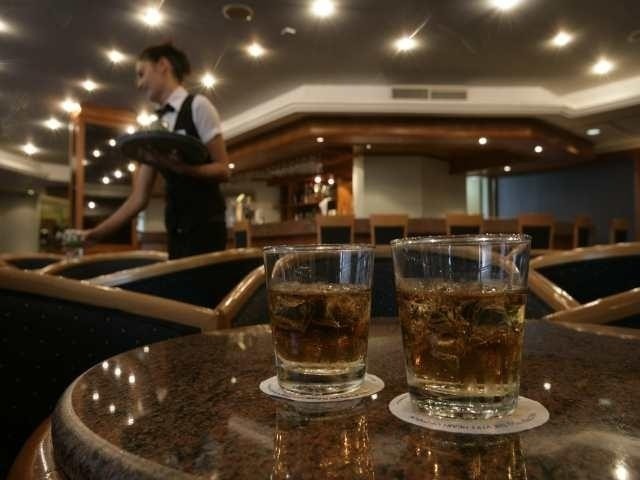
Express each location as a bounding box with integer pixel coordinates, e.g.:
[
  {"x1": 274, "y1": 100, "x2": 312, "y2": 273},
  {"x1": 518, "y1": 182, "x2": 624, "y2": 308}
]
[
  {"x1": 261, "y1": 243, "x2": 375, "y2": 393},
  {"x1": 151, "y1": 120, "x2": 169, "y2": 132},
  {"x1": 390, "y1": 234, "x2": 532, "y2": 421}
]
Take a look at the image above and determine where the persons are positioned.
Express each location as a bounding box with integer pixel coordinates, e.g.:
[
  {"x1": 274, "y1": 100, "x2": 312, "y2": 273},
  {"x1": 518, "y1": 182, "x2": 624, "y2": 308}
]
[{"x1": 61, "y1": 40, "x2": 231, "y2": 261}]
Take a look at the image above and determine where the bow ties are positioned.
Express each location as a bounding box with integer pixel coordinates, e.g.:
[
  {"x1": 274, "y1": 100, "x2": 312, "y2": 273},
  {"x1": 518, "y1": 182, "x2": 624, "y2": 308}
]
[{"x1": 154, "y1": 104, "x2": 175, "y2": 119}]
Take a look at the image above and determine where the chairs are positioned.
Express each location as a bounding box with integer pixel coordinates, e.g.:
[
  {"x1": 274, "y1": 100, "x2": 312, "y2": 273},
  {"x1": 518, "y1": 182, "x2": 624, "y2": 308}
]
[
  {"x1": 369, "y1": 213, "x2": 409, "y2": 244},
  {"x1": 316, "y1": 214, "x2": 355, "y2": 243},
  {"x1": 445, "y1": 215, "x2": 484, "y2": 236},
  {"x1": 574, "y1": 217, "x2": 594, "y2": 249},
  {"x1": 517, "y1": 215, "x2": 554, "y2": 247}
]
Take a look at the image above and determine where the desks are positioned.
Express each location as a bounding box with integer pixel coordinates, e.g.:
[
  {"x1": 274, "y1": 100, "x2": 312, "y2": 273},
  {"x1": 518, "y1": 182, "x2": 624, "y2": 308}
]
[{"x1": 41, "y1": 315, "x2": 640, "y2": 480}]
